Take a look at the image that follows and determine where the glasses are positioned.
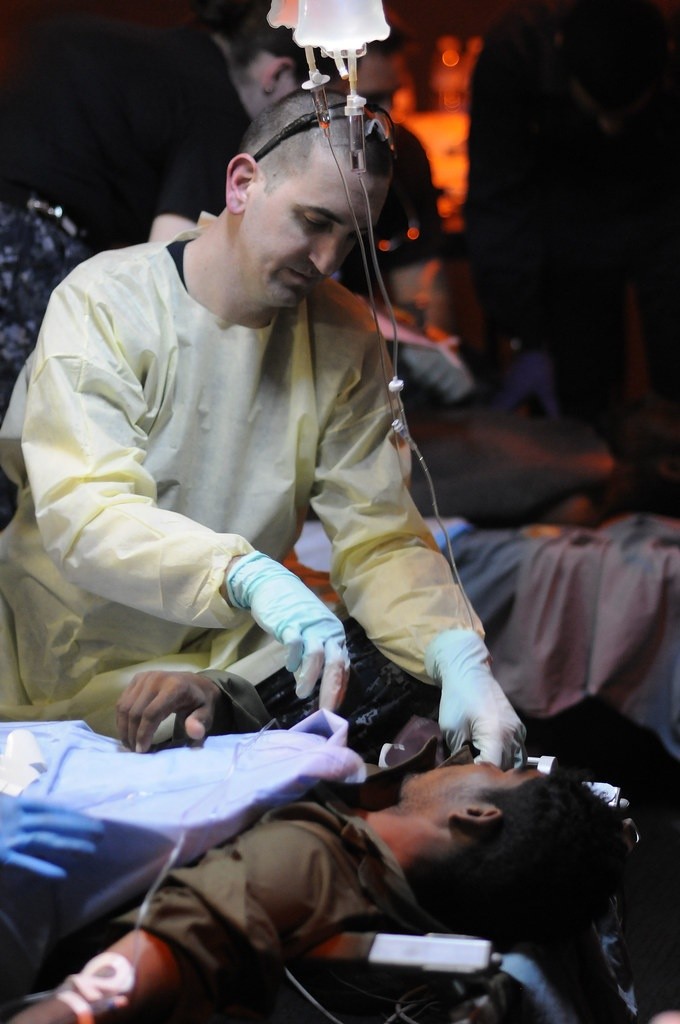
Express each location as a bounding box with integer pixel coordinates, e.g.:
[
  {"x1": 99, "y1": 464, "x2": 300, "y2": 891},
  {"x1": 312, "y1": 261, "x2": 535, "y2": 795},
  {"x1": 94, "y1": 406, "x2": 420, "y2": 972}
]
[{"x1": 253, "y1": 99, "x2": 398, "y2": 158}]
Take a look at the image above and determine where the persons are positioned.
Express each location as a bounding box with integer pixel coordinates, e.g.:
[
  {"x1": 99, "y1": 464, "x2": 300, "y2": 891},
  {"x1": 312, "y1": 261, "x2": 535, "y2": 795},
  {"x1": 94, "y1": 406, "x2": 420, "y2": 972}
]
[
  {"x1": 1, "y1": 0, "x2": 680, "y2": 536},
  {"x1": 0, "y1": 668, "x2": 634, "y2": 1024},
  {"x1": 149, "y1": 19, "x2": 473, "y2": 398},
  {"x1": 0, "y1": 85, "x2": 531, "y2": 772}
]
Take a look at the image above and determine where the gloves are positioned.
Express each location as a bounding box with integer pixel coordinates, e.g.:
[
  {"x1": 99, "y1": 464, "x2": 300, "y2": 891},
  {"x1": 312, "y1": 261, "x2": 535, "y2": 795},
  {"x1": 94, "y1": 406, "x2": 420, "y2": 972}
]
[
  {"x1": 224, "y1": 548, "x2": 352, "y2": 712},
  {"x1": 424, "y1": 629, "x2": 528, "y2": 772}
]
